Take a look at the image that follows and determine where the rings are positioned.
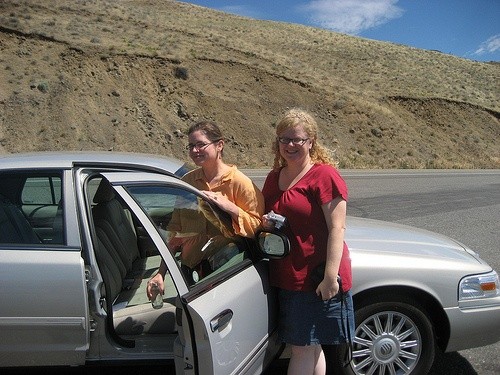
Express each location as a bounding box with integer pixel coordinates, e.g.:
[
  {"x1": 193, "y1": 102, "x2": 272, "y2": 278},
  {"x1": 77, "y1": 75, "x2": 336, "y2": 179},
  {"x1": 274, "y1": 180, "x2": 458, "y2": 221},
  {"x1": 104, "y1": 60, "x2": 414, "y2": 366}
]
[{"x1": 214, "y1": 195, "x2": 218, "y2": 199}]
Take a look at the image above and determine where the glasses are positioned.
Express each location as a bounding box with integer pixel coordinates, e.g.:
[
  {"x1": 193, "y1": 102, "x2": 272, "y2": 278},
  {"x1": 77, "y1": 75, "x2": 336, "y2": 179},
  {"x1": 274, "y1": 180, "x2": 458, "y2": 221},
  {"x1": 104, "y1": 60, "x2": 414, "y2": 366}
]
[
  {"x1": 277, "y1": 136, "x2": 310, "y2": 144},
  {"x1": 186, "y1": 138, "x2": 223, "y2": 150}
]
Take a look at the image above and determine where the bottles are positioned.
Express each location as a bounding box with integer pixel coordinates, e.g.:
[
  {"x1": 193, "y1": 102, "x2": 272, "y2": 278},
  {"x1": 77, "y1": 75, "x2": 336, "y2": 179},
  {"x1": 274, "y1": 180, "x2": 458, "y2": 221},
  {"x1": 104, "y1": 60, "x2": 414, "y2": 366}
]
[{"x1": 151, "y1": 283, "x2": 162, "y2": 309}]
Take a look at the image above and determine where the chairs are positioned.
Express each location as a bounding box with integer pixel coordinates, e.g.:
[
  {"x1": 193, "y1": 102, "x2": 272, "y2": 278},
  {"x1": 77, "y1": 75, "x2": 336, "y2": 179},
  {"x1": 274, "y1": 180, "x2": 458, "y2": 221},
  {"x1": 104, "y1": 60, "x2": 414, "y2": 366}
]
[
  {"x1": 91, "y1": 226, "x2": 180, "y2": 336},
  {"x1": 92, "y1": 178, "x2": 191, "y2": 276},
  {"x1": 1, "y1": 172, "x2": 46, "y2": 244}
]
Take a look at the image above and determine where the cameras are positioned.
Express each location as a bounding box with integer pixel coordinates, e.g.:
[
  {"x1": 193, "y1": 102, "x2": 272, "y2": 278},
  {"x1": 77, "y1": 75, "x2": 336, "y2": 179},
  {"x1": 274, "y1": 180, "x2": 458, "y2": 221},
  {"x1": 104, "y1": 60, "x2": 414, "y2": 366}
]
[{"x1": 268, "y1": 212, "x2": 288, "y2": 230}]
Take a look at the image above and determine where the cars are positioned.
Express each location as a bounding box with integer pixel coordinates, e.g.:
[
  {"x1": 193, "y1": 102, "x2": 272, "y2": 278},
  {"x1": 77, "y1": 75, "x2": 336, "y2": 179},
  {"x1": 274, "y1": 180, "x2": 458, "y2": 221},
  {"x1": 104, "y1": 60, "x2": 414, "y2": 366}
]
[{"x1": 0, "y1": 150, "x2": 500, "y2": 374}]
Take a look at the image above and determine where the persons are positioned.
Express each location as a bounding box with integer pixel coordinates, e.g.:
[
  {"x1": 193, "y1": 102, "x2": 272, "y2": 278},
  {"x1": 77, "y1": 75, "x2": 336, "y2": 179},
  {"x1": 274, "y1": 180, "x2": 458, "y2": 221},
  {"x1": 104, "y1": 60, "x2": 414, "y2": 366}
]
[
  {"x1": 146, "y1": 121, "x2": 266, "y2": 326},
  {"x1": 261, "y1": 110, "x2": 356, "y2": 375}
]
